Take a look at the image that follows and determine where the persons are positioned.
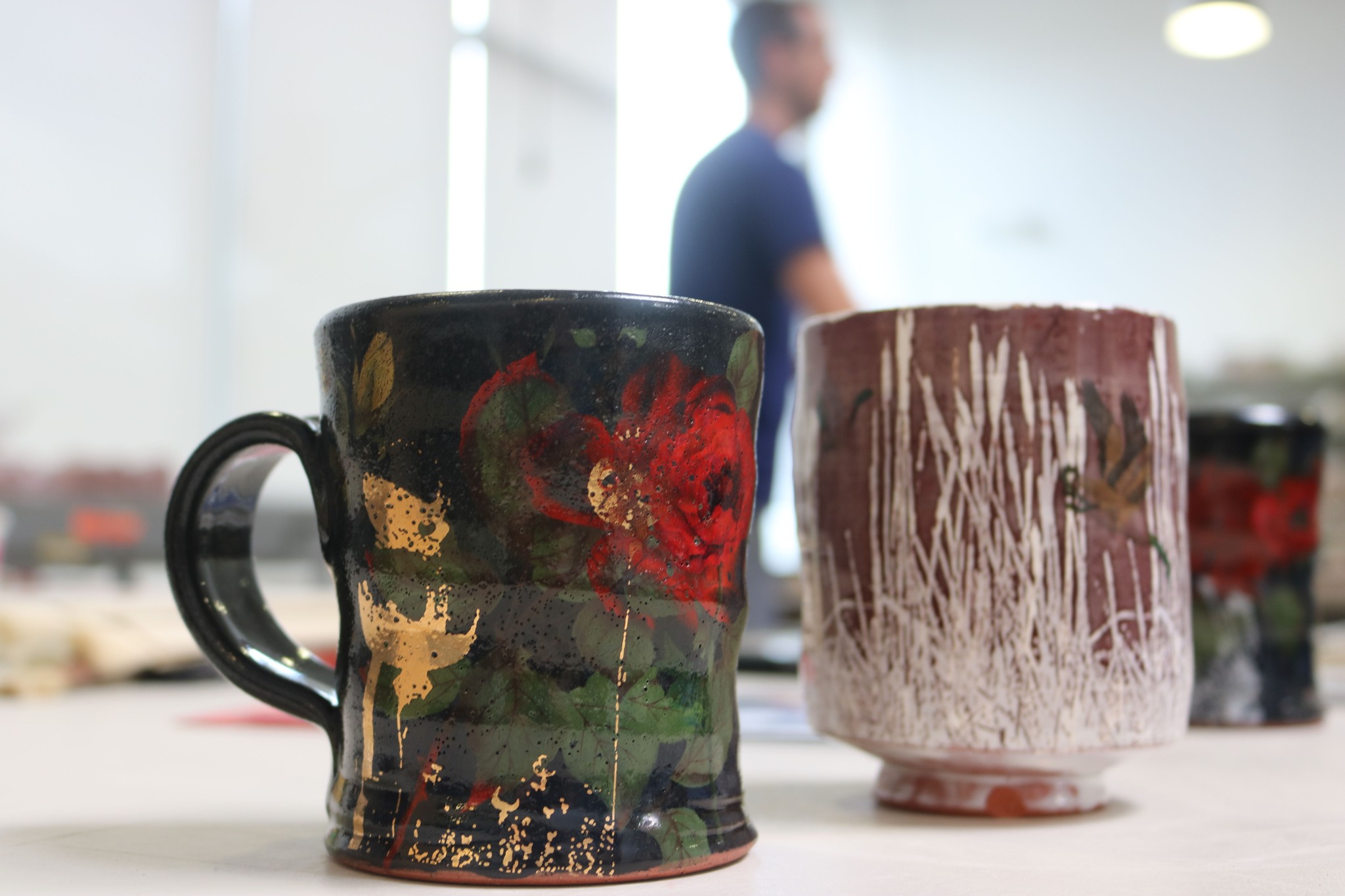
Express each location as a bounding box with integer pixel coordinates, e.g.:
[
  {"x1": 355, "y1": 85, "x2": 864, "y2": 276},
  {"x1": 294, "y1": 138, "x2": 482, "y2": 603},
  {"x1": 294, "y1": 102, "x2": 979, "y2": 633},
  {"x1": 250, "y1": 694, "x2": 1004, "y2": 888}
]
[{"x1": 668, "y1": 0, "x2": 860, "y2": 506}]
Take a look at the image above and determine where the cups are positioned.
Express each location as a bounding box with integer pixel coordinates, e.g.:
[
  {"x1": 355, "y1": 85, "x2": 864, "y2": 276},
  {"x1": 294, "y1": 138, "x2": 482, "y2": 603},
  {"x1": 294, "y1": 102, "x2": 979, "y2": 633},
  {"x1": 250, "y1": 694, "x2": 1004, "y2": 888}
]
[
  {"x1": 1186, "y1": 409, "x2": 1345, "y2": 729},
  {"x1": 164, "y1": 288, "x2": 766, "y2": 888},
  {"x1": 786, "y1": 300, "x2": 1196, "y2": 821}
]
